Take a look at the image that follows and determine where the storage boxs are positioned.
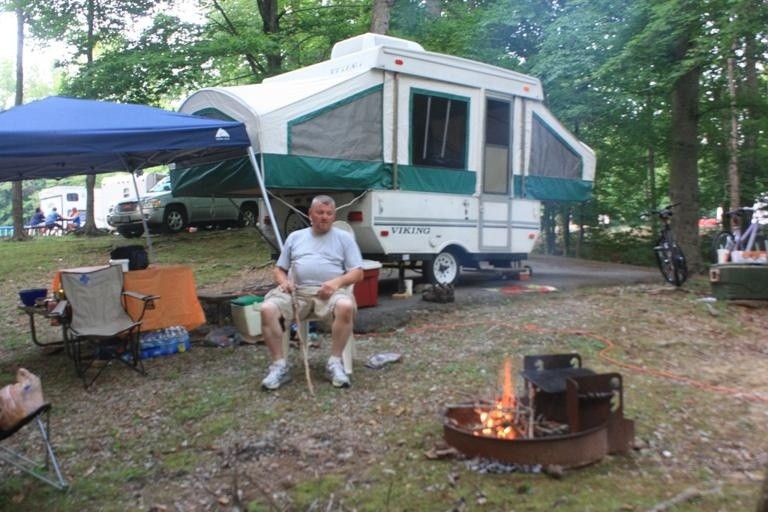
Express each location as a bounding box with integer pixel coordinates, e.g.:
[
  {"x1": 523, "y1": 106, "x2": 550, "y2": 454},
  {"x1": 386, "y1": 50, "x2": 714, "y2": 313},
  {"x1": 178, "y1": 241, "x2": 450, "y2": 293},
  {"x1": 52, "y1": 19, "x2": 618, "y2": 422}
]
[
  {"x1": 230, "y1": 294, "x2": 264, "y2": 337},
  {"x1": 353, "y1": 257, "x2": 383, "y2": 307}
]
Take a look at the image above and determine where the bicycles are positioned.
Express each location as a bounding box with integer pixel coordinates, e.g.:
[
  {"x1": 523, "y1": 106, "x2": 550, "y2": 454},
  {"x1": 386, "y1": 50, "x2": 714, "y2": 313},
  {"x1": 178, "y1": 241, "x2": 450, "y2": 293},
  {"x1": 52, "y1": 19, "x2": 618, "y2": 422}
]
[
  {"x1": 711, "y1": 207, "x2": 753, "y2": 263},
  {"x1": 637, "y1": 201, "x2": 688, "y2": 286}
]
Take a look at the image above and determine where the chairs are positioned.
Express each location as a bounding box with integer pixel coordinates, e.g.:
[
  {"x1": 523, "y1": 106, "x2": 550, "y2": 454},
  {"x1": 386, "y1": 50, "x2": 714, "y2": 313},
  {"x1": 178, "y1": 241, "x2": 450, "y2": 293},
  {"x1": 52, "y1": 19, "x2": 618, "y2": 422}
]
[
  {"x1": 281, "y1": 221, "x2": 355, "y2": 376},
  {"x1": 44, "y1": 263, "x2": 161, "y2": 392}
]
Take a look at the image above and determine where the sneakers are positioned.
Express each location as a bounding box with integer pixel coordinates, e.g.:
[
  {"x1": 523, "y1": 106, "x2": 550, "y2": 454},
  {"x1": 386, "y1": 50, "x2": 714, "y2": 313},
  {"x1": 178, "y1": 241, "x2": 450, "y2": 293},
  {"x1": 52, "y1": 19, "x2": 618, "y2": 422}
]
[
  {"x1": 325, "y1": 360, "x2": 351, "y2": 388},
  {"x1": 261, "y1": 363, "x2": 292, "y2": 389},
  {"x1": 422, "y1": 283, "x2": 455, "y2": 303}
]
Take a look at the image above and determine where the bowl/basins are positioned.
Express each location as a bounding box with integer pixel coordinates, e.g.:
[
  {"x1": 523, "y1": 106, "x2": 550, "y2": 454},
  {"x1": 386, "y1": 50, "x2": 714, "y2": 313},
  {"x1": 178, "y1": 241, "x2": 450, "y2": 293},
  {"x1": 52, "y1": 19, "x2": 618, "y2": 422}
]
[{"x1": 19, "y1": 289, "x2": 48, "y2": 307}]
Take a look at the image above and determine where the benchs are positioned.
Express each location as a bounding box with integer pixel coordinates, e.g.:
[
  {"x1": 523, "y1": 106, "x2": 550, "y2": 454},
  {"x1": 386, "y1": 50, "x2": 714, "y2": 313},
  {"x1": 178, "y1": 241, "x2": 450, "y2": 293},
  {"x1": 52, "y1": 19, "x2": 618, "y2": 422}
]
[
  {"x1": 18, "y1": 303, "x2": 72, "y2": 360},
  {"x1": 197, "y1": 289, "x2": 237, "y2": 329}
]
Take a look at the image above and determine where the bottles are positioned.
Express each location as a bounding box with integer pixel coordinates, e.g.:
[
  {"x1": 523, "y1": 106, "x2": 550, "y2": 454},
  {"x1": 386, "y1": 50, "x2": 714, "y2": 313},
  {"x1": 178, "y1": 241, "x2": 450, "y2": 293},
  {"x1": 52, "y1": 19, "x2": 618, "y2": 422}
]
[
  {"x1": 141, "y1": 326, "x2": 189, "y2": 357},
  {"x1": 716, "y1": 204, "x2": 723, "y2": 224}
]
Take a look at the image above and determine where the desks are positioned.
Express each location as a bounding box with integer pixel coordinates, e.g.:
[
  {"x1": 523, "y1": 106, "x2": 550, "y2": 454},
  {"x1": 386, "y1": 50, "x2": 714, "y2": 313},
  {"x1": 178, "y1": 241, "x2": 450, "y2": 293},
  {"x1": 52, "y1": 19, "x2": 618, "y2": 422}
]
[{"x1": 58, "y1": 265, "x2": 192, "y2": 360}]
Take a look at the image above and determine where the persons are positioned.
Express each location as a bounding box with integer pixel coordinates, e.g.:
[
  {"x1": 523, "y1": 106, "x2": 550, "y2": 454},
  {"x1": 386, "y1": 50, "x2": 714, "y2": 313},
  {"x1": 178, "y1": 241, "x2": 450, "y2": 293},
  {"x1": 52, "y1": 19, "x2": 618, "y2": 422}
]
[
  {"x1": 46, "y1": 208, "x2": 63, "y2": 237},
  {"x1": 29, "y1": 207, "x2": 48, "y2": 237},
  {"x1": 67, "y1": 207, "x2": 80, "y2": 231},
  {"x1": 260, "y1": 194, "x2": 364, "y2": 391}
]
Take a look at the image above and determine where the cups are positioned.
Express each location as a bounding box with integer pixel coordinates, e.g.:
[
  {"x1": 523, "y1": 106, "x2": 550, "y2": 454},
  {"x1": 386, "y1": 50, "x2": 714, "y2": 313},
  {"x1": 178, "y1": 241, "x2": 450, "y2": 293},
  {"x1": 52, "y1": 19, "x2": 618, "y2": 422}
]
[
  {"x1": 716, "y1": 249, "x2": 729, "y2": 265},
  {"x1": 404, "y1": 280, "x2": 413, "y2": 296}
]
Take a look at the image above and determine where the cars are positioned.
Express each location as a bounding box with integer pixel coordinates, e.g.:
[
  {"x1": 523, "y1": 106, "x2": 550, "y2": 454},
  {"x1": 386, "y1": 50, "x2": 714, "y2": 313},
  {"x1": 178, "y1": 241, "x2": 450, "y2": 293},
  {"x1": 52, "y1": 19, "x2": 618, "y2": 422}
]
[{"x1": 107, "y1": 176, "x2": 259, "y2": 238}]
